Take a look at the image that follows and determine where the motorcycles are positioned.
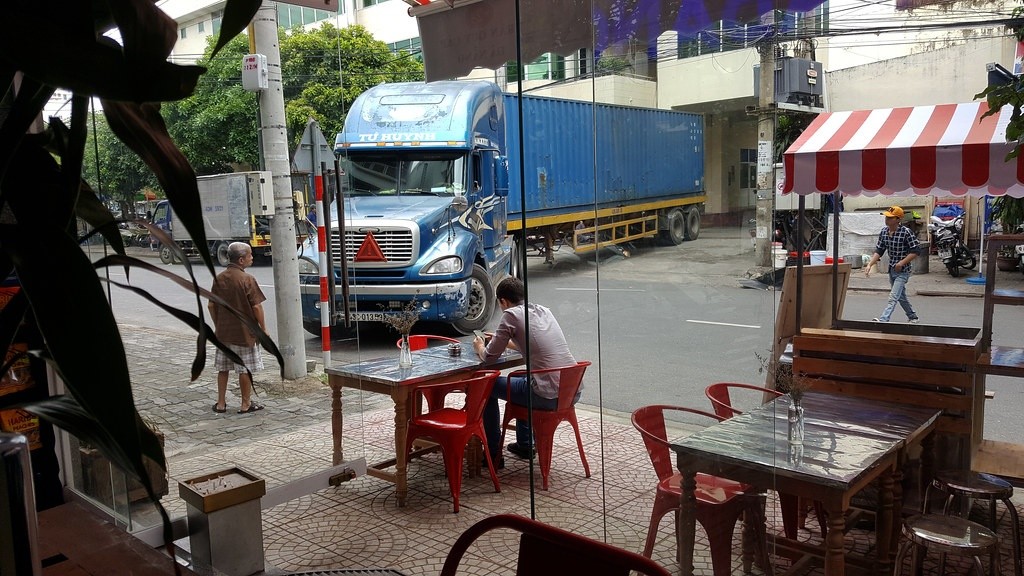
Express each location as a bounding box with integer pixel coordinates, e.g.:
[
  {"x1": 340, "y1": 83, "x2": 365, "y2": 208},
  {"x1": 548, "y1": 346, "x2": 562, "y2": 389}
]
[
  {"x1": 926, "y1": 210, "x2": 977, "y2": 277},
  {"x1": 117, "y1": 223, "x2": 152, "y2": 248}
]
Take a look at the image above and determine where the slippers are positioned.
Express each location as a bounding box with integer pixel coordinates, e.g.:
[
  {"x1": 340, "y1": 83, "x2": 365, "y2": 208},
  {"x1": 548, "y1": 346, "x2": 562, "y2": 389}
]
[
  {"x1": 237, "y1": 400, "x2": 263, "y2": 413},
  {"x1": 212, "y1": 402, "x2": 227, "y2": 413}
]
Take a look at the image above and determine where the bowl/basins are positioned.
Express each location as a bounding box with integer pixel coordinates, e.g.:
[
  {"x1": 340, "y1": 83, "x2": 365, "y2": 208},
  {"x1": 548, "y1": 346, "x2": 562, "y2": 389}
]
[{"x1": 826, "y1": 258, "x2": 843, "y2": 264}]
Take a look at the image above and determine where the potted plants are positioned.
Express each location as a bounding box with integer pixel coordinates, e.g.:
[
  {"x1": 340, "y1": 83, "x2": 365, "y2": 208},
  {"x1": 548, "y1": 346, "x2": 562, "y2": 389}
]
[{"x1": 993, "y1": 198, "x2": 1020, "y2": 271}]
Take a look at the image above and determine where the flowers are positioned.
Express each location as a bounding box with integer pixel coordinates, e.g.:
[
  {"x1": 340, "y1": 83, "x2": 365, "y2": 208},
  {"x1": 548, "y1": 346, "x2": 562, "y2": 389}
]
[
  {"x1": 376, "y1": 289, "x2": 421, "y2": 333},
  {"x1": 754, "y1": 352, "x2": 823, "y2": 402}
]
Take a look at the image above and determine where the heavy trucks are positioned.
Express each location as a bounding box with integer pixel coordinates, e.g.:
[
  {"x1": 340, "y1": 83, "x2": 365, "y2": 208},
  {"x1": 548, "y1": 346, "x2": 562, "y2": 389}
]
[{"x1": 293, "y1": 64, "x2": 709, "y2": 339}]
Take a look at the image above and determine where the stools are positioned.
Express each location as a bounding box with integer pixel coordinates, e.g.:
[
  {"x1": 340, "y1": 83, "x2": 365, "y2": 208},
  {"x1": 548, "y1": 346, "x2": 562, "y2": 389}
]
[
  {"x1": 922, "y1": 471, "x2": 1023, "y2": 576},
  {"x1": 893, "y1": 513, "x2": 1000, "y2": 576}
]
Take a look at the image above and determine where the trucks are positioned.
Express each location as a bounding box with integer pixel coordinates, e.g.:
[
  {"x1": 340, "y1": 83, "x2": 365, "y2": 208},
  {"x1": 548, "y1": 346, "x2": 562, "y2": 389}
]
[{"x1": 145, "y1": 168, "x2": 278, "y2": 269}]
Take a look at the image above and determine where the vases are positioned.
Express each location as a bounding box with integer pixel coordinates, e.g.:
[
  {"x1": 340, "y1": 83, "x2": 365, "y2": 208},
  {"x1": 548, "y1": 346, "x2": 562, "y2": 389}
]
[
  {"x1": 789, "y1": 399, "x2": 805, "y2": 446},
  {"x1": 398, "y1": 331, "x2": 413, "y2": 368}
]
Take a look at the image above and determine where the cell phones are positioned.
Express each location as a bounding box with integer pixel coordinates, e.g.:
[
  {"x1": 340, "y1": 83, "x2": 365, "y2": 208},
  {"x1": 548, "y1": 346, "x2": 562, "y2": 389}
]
[{"x1": 473, "y1": 330, "x2": 489, "y2": 347}]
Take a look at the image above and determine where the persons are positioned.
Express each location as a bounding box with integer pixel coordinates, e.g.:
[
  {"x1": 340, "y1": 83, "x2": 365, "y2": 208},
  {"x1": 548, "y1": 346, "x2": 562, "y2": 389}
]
[
  {"x1": 146, "y1": 210, "x2": 152, "y2": 224},
  {"x1": 865, "y1": 206, "x2": 920, "y2": 324},
  {"x1": 208, "y1": 243, "x2": 270, "y2": 413},
  {"x1": 474, "y1": 277, "x2": 585, "y2": 468}
]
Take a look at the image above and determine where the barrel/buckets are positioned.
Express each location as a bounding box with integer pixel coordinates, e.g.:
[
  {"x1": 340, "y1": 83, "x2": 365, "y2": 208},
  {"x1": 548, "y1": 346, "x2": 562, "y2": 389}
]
[
  {"x1": 771, "y1": 241, "x2": 787, "y2": 268},
  {"x1": 810, "y1": 250, "x2": 826, "y2": 265}
]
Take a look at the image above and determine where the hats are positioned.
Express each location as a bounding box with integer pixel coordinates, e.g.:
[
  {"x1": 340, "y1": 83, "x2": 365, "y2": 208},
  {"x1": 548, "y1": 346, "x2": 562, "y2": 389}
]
[{"x1": 880, "y1": 206, "x2": 904, "y2": 220}]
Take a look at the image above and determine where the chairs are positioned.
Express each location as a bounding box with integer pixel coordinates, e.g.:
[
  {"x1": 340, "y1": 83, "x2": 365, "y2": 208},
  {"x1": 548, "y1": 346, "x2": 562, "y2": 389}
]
[
  {"x1": 439, "y1": 513, "x2": 670, "y2": 576},
  {"x1": 630, "y1": 405, "x2": 766, "y2": 576},
  {"x1": 705, "y1": 383, "x2": 827, "y2": 545},
  {"x1": 395, "y1": 334, "x2": 593, "y2": 512}
]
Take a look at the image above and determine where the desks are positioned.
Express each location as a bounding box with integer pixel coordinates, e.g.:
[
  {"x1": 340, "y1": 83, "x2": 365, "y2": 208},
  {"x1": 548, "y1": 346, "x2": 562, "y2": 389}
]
[
  {"x1": 324, "y1": 342, "x2": 527, "y2": 509},
  {"x1": 666, "y1": 391, "x2": 950, "y2": 576}
]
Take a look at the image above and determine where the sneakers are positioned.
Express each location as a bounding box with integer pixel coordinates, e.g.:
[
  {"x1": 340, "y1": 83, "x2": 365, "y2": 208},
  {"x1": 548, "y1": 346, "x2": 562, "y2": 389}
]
[
  {"x1": 507, "y1": 443, "x2": 536, "y2": 458},
  {"x1": 907, "y1": 318, "x2": 920, "y2": 324},
  {"x1": 482, "y1": 452, "x2": 504, "y2": 468}
]
